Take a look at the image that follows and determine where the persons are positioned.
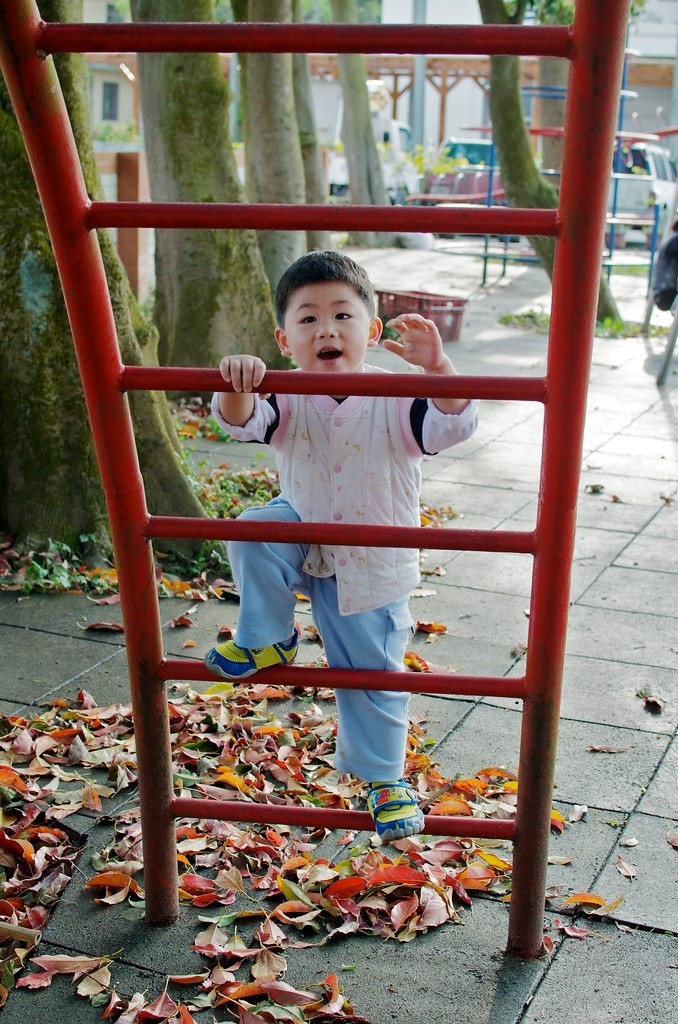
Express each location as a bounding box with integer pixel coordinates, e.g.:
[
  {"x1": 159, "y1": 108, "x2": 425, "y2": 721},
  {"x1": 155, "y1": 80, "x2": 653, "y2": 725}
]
[
  {"x1": 655, "y1": 220, "x2": 678, "y2": 312},
  {"x1": 204, "y1": 250, "x2": 477, "y2": 840}
]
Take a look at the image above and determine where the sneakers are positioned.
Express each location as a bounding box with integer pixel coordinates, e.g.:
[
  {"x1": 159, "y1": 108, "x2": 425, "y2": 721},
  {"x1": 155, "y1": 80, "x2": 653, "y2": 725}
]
[
  {"x1": 204, "y1": 625, "x2": 299, "y2": 679},
  {"x1": 365, "y1": 778, "x2": 425, "y2": 841}
]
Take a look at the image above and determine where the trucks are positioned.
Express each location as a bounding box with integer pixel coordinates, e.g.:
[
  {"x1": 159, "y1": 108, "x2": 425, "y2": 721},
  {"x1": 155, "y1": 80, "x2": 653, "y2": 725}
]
[{"x1": 296, "y1": 82, "x2": 420, "y2": 206}]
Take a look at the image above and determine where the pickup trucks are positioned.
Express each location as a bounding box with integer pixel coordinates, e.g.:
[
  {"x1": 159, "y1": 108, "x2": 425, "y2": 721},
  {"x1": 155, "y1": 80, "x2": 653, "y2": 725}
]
[{"x1": 423, "y1": 140, "x2": 522, "y2": 243}]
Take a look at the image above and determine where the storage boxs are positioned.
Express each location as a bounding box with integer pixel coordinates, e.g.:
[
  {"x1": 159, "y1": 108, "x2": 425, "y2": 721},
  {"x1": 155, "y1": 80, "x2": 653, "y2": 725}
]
[{"x1": 373, "y1": 290, "x2": 466, "y2": 341}]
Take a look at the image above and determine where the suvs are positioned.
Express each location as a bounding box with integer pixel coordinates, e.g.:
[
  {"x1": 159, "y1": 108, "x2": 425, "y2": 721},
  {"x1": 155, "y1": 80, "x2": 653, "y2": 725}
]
[{"x1": 610, "y1": 145, "x2": 676, "y2": 230}]
